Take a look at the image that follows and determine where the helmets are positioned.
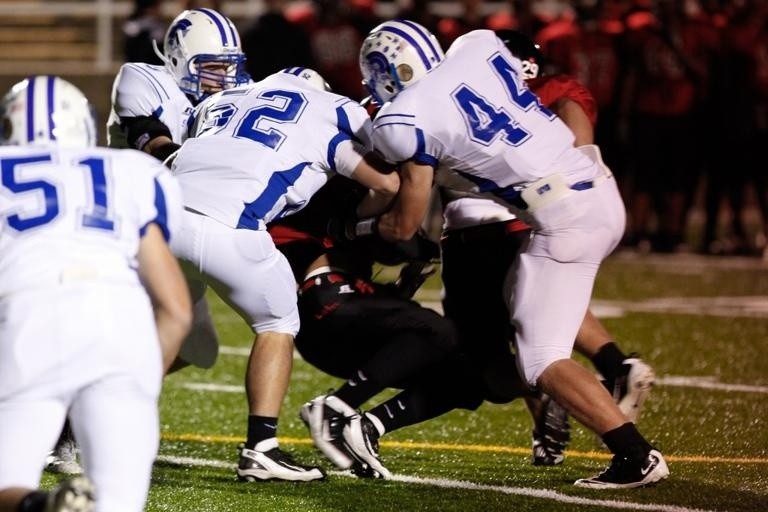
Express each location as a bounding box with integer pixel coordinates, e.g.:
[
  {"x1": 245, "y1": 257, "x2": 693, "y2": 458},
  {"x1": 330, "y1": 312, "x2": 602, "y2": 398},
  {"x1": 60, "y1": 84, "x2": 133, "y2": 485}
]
[
  {"x1": 495, "y1": 29, "x2": 548, "y2": 80},
  {"x1": 0, "y1": 74, "x2": 98, "y2": 145},
  {"x1": 276, "y1": 66, "x2": 332, "y2": 92},
  {"x1": 358, "y1": 20, "x2": 446, "y2": 107},
  {"x1": 164, "y1": 8, "x2": 249, "y2": 102}
]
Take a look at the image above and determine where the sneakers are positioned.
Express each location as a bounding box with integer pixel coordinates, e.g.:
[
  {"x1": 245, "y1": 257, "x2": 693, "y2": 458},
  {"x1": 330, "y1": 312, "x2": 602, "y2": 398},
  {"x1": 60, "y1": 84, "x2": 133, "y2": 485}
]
[
  {"x1": 236, "y1": 389, "x2": 392, "y2": 482},
  {"x1": 45, "y1": 444, "x2": 95, "y2": 512},
  {"x1": 533, "y1": 352, "x2": 669, "y2": 490}
]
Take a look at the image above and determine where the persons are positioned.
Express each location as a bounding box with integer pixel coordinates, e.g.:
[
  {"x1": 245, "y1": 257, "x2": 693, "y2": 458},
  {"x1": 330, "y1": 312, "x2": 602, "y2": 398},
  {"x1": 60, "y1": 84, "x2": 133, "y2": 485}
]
[
  {"x1": 0, "y1": 77, "x2": 194, "y2": 511},
  {"x1": 43, "y1": 1, "x2": 767, "y2": 493}
]
